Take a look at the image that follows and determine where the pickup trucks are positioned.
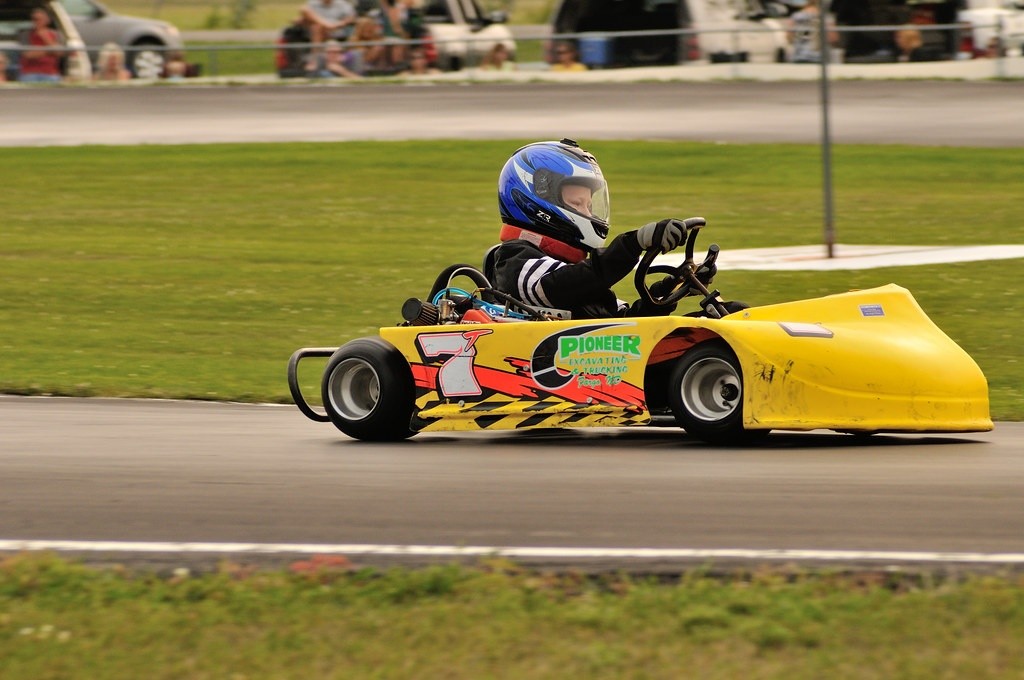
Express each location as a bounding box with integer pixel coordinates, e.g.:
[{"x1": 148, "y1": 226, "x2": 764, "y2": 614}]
[
  {"x1": 547, "y1": 0, "x2": 806, "y2": 65},
  {"x1": 275, "y1": 0, "x2": 516, "y2": 79}
]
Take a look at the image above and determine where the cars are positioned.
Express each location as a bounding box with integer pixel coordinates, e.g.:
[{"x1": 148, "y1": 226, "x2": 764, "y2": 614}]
[
  {"x1": 822, "y1": 0, "x2": 1024, "y2": 63},
  {"x1": 0, "y1": 0, "x2": 184, "y2": 80}
]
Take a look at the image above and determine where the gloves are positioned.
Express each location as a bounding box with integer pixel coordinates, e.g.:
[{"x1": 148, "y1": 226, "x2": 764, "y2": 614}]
[
  {"x1": 622, "y1": 217, "x2": 706, "y2": 255},
  {"x1": 652, "y1": 264, "x2": 718, "y2": 296}
]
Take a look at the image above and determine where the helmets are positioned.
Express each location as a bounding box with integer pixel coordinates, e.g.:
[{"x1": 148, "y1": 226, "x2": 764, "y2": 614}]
[{"x1": 497, "y1": 140, "x2": 611, "y2": 251}]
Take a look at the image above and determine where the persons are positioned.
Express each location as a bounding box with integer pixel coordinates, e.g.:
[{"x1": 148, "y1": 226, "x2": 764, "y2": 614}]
[
  {"x1": 785, "y1": 0, "x2": 839, "y2": 64},
  {"x1": 832, "y1": 0, "x2": 1005, "y2": 65},
  {"x1": 0, "y1": 10, "x2": 184, "y2": 83},
  {"x1": 548, "y1": 0, "x2": 688, "y2": 73},
  {"x1": 484, "y1": 42, "x2": 515, "y2": 73},
  {"x1": 275, "y1": 1, "x2": 441, "y2": 76},
  {"x1": 493, "y1": 138, "x2": 720, "y2": 319}
]
